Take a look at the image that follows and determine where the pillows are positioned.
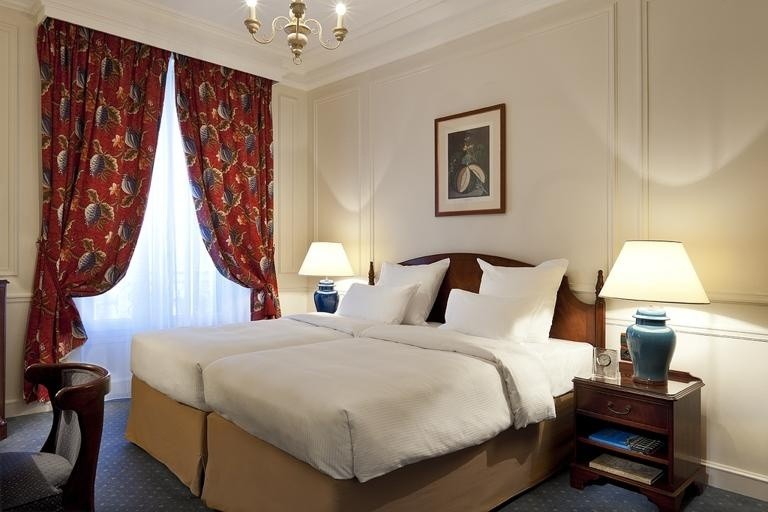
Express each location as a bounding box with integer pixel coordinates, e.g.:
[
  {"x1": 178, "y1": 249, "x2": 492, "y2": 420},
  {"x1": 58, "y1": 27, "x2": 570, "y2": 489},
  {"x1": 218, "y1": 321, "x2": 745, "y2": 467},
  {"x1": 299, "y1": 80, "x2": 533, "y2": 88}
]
[
  {"x1": 446, "y1": 288, "x2": 532, "y2": 349},
  {"x1": 377, "y1": 258, "x2": 450, "y2": 325},
  {"x1": 334, "y1": 282, "x2": 421, "y2": 325},
  {"x1": 475, "y1": 257, "x2": 569, "y2": 357}
]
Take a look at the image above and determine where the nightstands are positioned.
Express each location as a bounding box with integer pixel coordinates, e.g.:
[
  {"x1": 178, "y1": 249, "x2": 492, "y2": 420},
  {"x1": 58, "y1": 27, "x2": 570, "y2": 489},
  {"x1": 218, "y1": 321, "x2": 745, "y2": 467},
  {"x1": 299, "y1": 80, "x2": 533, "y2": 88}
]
[{"x1": 568, "y1": 364, "x2": 707, "y2": 512}]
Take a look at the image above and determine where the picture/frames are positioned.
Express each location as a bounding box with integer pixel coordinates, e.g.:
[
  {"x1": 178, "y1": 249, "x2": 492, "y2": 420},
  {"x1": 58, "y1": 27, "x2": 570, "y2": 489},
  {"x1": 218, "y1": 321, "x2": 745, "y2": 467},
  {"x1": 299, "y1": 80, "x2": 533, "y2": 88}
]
[{"x1": 434, "y1": 103, "x2": 506, "y2": 216}]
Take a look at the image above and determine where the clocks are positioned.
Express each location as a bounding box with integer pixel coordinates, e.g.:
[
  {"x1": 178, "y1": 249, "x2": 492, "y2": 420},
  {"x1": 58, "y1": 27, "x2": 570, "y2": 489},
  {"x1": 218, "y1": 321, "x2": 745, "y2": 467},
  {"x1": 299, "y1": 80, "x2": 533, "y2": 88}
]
[{"x1": 592, "y1": 346, "x2": 619, "y2": 384}]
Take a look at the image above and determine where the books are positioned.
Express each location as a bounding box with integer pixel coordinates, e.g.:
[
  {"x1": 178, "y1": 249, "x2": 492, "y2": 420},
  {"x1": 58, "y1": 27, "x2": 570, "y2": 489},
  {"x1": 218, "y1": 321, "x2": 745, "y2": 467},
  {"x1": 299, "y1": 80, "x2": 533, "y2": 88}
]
[
  {"x1": 588, "y1": 452, "x2": 665, "y2": 485},
  {"x1": 589, "y1": 427, "x2": 661, "y2": 456}
]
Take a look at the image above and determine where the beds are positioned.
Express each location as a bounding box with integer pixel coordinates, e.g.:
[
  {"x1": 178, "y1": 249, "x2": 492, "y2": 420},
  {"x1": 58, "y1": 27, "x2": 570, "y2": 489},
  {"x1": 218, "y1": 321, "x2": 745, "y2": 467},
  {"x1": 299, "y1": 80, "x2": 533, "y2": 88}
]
[{"x1": 126, "y1": 251, "x2": 604, "y2": 511}]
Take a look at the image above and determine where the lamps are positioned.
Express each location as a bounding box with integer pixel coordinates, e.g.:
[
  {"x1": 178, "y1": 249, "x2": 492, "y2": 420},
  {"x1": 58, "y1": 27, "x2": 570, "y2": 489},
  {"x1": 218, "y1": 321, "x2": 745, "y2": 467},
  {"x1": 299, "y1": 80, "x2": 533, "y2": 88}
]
[
  {"x1": 243, "y1": 0, "x2": 347, "y2": 65},
  {"x1": 297, "y1": 242, "x2": 354, "y2": 314},
  {"x1": 596, "y1": 239, "x2": 710, "y2": 385}
]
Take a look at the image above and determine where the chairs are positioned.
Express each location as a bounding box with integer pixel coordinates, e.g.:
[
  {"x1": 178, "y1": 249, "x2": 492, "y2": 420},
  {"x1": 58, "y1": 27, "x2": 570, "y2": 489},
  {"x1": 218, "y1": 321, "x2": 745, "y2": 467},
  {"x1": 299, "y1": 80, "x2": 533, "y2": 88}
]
[{"x1": 0, "y1": 361, "x2": 111, "y2": 512}]
